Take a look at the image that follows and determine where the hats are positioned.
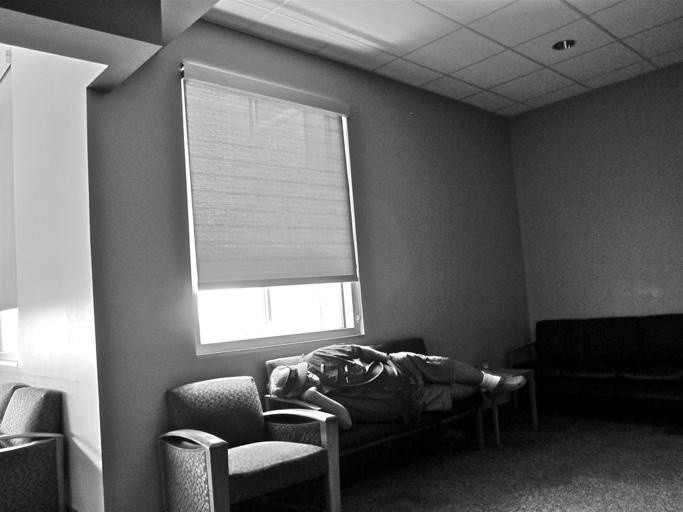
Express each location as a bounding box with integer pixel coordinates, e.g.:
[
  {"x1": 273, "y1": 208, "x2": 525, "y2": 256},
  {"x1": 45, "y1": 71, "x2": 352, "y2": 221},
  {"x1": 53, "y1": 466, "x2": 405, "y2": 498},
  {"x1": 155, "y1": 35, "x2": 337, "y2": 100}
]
[{"x1": 276, "y1": 362, "x2": 308, "y2": 398}]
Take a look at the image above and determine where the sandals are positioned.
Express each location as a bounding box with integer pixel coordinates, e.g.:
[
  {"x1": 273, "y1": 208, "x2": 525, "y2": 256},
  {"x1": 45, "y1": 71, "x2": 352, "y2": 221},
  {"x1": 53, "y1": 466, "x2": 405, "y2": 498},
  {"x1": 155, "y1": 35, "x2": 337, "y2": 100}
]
[{"x1": 483, "y1": 374, "x2": 527, "y2": 398}]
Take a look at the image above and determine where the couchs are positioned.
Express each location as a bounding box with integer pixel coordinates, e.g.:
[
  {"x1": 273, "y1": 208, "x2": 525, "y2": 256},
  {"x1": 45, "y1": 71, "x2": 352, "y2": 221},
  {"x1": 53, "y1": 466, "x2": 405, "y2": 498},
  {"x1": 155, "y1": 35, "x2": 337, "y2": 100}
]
[
  {"x1": 265, "y1": 338, "x2": 536, "y2": 483},
  {"x1": 508, "y1": 312, "x2": 682, "y2": 425},
  {"x1": 0, "y1": 381, "x2": 68, "y2": 511}
]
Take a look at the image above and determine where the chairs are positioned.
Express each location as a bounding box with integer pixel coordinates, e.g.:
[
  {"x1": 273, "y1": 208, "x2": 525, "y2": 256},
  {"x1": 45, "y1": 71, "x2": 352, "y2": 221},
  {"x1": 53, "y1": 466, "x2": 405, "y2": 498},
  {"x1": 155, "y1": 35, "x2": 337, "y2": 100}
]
[{"x1": 157, "y1": 376, "x2": 343, "y2": 512}]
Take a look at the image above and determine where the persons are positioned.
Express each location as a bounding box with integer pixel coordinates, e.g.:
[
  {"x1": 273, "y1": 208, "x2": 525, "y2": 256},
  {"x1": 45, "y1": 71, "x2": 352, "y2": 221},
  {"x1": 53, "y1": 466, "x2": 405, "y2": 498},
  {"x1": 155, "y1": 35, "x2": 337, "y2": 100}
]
[{"x1": 267, "y1": 342, "x2": 528, "y2": 430}]
